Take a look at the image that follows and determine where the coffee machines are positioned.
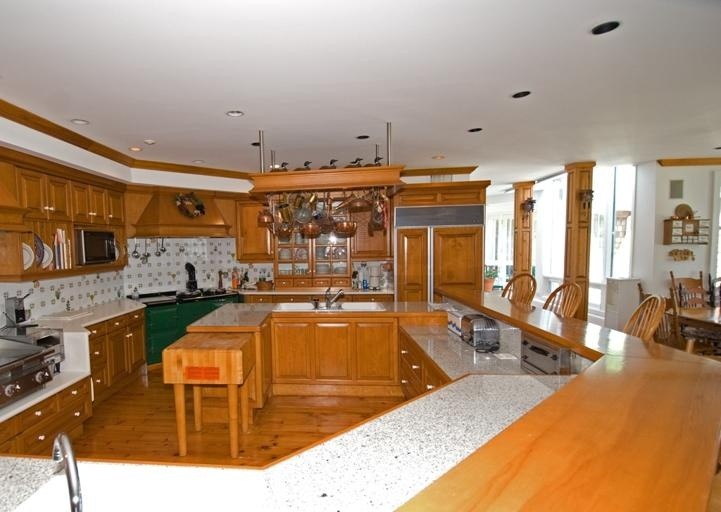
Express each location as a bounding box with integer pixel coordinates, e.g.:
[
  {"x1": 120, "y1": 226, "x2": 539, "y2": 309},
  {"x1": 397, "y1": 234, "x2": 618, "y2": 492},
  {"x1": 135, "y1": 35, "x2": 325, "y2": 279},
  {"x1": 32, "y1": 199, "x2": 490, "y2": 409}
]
[{"x1": 368, "y1": 265, "x2": 382, "y2": 290}]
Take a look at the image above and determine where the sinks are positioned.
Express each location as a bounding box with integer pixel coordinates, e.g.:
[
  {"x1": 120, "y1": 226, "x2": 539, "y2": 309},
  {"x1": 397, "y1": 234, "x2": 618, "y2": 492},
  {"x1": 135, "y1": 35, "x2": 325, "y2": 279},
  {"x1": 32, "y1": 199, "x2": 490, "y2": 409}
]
[{"x1": 342, "y1": 301, "x2": 387, "y2": 312}]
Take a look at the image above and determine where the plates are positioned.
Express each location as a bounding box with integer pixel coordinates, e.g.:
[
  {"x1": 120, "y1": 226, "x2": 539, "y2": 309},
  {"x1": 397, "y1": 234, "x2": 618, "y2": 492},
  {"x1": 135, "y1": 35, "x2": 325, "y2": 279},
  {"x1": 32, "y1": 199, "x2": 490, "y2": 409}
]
[
  {"x1": 676, "y1": 205, "x2": 694, "y2": 220},
  {"x1": 335, "y1": 247, "x2": 346, "y2": 259},
  {"x1": 34, "y1": 233, "x2": 44, "y2": 268},
  {"x1": 43, "y1": 244, "x2": 53, "y2": 268},
  {"x1": 296, "y1": 247, "x2": 308, "y2": 260},
  {"x1": 22, "y1": 243, "x2": 34, "y2": 270},
  {"x1": 279, "y1": 248, "x2": 291, "y2": 260}
]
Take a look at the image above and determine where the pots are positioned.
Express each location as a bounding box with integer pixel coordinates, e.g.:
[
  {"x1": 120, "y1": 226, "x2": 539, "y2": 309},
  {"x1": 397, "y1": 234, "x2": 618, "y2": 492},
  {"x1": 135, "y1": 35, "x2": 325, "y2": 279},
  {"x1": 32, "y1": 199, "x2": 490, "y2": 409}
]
[{"x1": 257, "y1": 189, "x2": 390, "y2": 237}]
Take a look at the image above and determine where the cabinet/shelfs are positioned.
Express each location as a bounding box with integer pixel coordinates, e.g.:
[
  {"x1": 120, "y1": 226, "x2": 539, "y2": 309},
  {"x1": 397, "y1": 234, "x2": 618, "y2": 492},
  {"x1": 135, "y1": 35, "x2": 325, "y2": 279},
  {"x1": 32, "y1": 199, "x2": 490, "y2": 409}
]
[
  {"x1": 0, "y1": 218, "x2": 77, "y2": 283},
  {"x1": 87, "y1": 308, "x2": 147, "y2": 398},
  {"x1": 110, "y1": 189, "x2": 127, "y2": 227},
  {"x1": 235, "y1": 197, "x2": 275, "y2": 263},
  {"x1": 242, "y1": 292, "x2": 394, "y2": 303},
  {"x1": 69, "y1": 181, "x2": 110, "y2": 226},
  {"x1": 0, "y1": 375, "x2": 93, "y2": 456},
  {"x1": 662, "y1": 217, "x2": 711, "y2": 246},
  {"x1": 350, "y1": 205, "x2": 391, "y2": 261},
  {"x1": 274, "y1": 200, "x2": 351, "y2": 290},
  {"x1": 0, "y1": 161, "x2": 23, "y2": 211},
  {"x1": 393, "y1": 180, "x2": 492, "y2": 303},
  {"x1": 15, "y1": 168, "x2": 73, "y2": 223},
  {"x1": 396, "y1": 328, "x2": 451, "y2": 400}
]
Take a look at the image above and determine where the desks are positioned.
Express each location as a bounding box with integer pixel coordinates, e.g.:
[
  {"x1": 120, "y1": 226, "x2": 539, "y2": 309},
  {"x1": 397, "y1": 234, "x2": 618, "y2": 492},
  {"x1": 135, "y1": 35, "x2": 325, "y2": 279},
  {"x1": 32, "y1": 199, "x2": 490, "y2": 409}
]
[{"x1": 162, "y1": 333, "x2": 257, "y2": 459}]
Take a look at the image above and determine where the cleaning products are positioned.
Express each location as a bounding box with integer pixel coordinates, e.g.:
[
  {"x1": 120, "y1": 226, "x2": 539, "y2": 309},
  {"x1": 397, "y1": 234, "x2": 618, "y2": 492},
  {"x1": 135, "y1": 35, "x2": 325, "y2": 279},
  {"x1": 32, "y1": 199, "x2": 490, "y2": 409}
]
[
  {"x1": 231, "y1": 266, "x2": 239, "y2": 289},
  {"x1": 132, "y1": 288, "x2": 139, "y2": 301}
]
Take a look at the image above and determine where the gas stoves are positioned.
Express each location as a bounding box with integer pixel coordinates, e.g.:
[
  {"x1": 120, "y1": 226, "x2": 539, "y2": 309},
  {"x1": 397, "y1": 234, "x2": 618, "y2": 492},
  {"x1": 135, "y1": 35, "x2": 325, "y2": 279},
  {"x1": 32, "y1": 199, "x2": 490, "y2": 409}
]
[{"x1": 0, "y1": 337, "x2": 58, "y2": 408}]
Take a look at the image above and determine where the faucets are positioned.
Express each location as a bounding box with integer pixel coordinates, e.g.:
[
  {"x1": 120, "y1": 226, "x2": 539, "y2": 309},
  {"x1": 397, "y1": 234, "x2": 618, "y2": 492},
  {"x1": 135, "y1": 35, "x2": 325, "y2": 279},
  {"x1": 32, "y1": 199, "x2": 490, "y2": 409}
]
[
  {"x1": 51, "y1": 430, "x2": 83, "y2": 512},
  {"x1": 325, "y1": 287, "x2": 346, "y2": 310}
]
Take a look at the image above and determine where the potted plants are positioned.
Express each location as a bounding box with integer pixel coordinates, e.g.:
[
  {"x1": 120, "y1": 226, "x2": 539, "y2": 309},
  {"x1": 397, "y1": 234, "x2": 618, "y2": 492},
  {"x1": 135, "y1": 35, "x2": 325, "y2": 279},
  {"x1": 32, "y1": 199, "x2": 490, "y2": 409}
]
[
  {"x1": 520, "y1": 196, "x2": 536, "y2": 215},
  {"x1": 484, "y1": 269, "x2": 498, "y2": 292}
]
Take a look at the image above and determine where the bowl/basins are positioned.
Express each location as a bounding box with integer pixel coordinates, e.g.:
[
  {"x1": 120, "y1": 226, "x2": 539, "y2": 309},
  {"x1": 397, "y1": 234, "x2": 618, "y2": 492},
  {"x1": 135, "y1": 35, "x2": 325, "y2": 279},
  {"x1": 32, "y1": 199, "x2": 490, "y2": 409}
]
[
  {"x1": 316, "y1": 264, "x2": 330, "y2": 273},
  {"x1": 257, "y1": 281, "x2": 274, "y2": 290},
  {"x1": 334, "y1": 266, "x2": 348, "y2": 274}
]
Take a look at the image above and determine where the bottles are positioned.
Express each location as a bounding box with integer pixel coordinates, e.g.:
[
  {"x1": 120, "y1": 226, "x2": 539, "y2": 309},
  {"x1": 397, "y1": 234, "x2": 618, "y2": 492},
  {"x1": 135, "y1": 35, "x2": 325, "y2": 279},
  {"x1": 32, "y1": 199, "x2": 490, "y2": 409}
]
[
  {"x1": 259, "y1": 271, "x2": 267, "y2": 282},
  {"x1": 232, "y1": 265, "x2": 239, "y2": 289},
  {"x1": 352, "y1": 268, "x2": 368, "y2": 289},
  {"x1": 218, "y1": 271, "x2": 222, "y2": 289}
]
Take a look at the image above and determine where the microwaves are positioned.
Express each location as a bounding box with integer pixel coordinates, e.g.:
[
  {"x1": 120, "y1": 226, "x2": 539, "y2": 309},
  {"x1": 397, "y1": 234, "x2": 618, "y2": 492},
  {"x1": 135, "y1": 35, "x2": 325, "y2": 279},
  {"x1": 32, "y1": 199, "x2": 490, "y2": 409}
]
[{"x1": 75, "y1": 229, "x2": 115, "y2": 265}]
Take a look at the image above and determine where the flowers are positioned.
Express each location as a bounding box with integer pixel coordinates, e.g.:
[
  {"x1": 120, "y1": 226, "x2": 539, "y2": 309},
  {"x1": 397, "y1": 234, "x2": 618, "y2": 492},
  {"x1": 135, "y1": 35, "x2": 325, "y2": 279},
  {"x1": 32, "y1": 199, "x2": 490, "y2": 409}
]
[{"x1": 174, "y1": 190, "x2": 207, "y2": 220}]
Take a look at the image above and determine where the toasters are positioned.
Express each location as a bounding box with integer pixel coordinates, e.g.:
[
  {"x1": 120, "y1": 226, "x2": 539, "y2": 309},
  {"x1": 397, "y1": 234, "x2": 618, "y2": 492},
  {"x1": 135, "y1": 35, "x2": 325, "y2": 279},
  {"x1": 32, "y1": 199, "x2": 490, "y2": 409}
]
[{"x1": 460, "y1": 314, "x2": 500, "y2": 353}]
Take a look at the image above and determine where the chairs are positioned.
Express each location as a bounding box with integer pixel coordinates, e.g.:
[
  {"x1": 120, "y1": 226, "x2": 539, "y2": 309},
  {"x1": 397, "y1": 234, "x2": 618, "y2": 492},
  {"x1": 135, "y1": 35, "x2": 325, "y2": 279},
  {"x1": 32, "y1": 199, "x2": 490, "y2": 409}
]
[
  {"x1": 625, "y1": 293, "x2": 668, "y2": 342},
  {"x1": 544, "y1": 282, "x2": 584, "y2": 320},
  {"x1": 637, "y1": 270, "x2": 720, "y2": 359},
  {"x1": 500, "y1": 272, "x2": 538, "y2": 306}
]
